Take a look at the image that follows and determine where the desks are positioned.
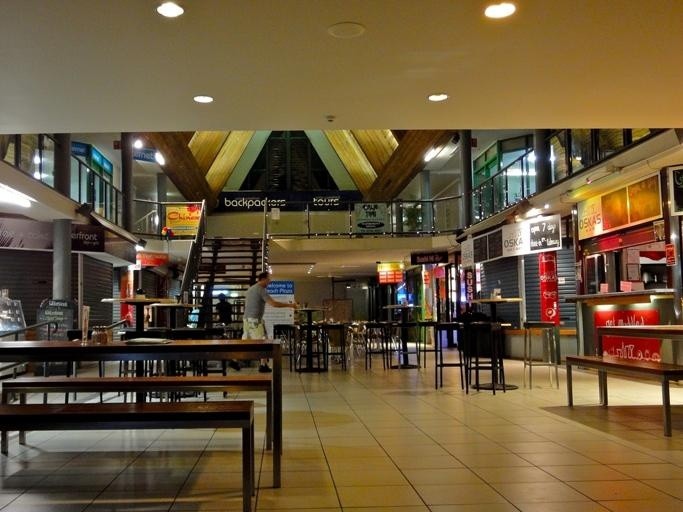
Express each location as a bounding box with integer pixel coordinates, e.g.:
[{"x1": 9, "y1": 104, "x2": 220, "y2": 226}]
[
  {"x1": 293, "y1": 306, "x2": 329, "y2": 373},
  {"x1": 471, "y1": 294, "x2": 523, "y2": 391},
  {"x1": 0, "y1": 340, "x2": 285, "y2": 512},
  {"x1": 384, "y1": 304, "x2": 422, "y2": 372},
  {"x1": 564, "y1": 322, "x2": 682, "y2": 439},
  {"x1": 101, "y1": 297, "x2": 202, "y2": 401}
]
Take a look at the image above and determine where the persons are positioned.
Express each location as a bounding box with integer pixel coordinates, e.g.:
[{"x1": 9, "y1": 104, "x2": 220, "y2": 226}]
[
  {"x1": 228, "y1": 272, "x2": 297, "y2": 373},
  {"x1": 214, "y1": 294, "x2": 232, "y2": 326}
]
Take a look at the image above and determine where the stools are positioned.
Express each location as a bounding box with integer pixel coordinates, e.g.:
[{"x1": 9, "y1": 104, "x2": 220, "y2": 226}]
[{"x1": 278, "y1": 319, "x2": 558, "y2": 394}]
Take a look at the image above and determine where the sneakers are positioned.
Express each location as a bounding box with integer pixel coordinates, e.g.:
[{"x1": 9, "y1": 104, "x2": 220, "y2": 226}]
[
  {"x1": 228, "y1": 359, "x2": 240, "y2": 371},
  {"x1": 259, "y1": 364, "x2": 272, "y2": 373}
]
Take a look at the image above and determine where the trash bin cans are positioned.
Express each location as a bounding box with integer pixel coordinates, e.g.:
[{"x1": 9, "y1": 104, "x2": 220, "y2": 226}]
[{"x1": 36, "y1": 298, "x2": 77, "y2": 376}]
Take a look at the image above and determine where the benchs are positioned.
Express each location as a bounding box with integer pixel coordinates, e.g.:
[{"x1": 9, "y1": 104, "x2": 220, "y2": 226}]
[
  {"x1": 567, "y1": 354, "x2": 682, "y2": 436},
  {"x1": 2, "y1": 374, "x2": 273, "y2": 512}
]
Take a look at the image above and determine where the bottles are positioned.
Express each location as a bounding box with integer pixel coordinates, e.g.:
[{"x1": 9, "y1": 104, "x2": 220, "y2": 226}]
[
  {"x1": 91, "y1": 325, "x2": 100, "y2": 344},
  {"x1": 100, "y1": 326, "x2": 109, "y2": 345}
]
